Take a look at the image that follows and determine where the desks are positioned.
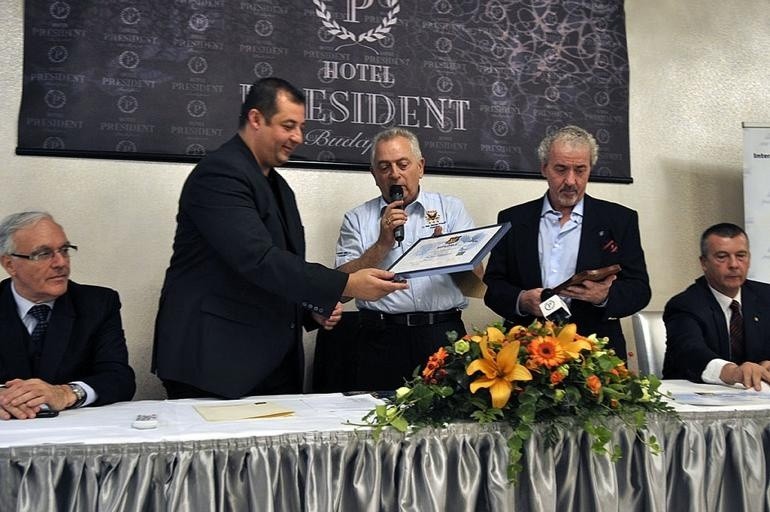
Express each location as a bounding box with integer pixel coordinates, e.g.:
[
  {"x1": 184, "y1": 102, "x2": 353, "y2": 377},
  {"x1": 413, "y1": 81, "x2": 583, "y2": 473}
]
[{"x1": 0, "y1": 376, "x2": 769, "y2": 512}]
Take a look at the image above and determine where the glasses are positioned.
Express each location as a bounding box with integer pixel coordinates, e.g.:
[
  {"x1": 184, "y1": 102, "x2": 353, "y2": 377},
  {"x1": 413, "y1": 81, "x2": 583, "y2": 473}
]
[{"x1": 10, "y1": 244, "x2": 78, "y2": 260}]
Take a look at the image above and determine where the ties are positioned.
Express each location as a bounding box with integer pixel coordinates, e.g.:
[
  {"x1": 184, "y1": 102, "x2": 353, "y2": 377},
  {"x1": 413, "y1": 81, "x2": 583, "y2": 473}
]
[
  {"x1": 730, "y1": 301, "x2": 745, "y2": 366},
  {"x1": 27, "y1": 304, "x2": 50, "y2": 341}
]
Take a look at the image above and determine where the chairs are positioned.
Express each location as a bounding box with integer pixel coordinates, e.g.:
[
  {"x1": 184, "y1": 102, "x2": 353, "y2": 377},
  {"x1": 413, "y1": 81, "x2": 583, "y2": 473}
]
[
  {"x1": 306, "y1": 311, "x2": 358, "y2": 394},
  {"x1": 631, "y1": 309, "x2": 667, "y2": 380}
]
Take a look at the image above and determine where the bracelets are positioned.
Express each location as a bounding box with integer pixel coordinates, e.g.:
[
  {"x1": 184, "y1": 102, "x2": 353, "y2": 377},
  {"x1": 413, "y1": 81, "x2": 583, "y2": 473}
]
[{"x1": 72, "y1": 385, "x2": 85, "y2": 401}]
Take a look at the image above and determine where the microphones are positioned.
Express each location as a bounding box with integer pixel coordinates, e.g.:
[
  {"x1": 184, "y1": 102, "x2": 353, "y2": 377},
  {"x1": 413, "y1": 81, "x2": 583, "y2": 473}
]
[
  {"x1": 387, "y1": 185, "x2": 406, "y2": 243},
  {"x1": 538, "y1": 286, "x2": 572, "y2": 326}
]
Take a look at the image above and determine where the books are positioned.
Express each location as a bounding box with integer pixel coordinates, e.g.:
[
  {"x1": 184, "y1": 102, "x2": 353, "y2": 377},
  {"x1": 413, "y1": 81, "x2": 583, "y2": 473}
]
[{"x1": 385, "y1": 221, "x2": 512, "y2": 280}]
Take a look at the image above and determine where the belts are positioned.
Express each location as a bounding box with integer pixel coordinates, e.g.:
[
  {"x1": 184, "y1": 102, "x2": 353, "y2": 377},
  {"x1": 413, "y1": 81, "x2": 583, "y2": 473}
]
[{"x1": 360, "y1": 306, "x2": 462, "y2": 326}]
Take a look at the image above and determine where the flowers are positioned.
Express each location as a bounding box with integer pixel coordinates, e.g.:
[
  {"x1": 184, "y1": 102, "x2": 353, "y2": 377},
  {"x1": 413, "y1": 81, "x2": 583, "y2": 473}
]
[{"x1": 341, "y1": 319, "x2": 688, "y2": 491}]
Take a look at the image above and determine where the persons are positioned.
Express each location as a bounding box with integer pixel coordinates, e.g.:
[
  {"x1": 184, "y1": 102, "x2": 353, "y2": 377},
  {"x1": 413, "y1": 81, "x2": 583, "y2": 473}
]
[
  {"x1": 0, "y1": 210, "x2": 136, "y2": 421},
  {"x1": 482, "y1": 125, "x2": 652, "y2": 369},
  {"x1": 150, "y1": 77, "x2": 410, "y2": 401},
  {"x1": 334, "y1": 128, "x2": 488, "y2": 392},
  {"x1": 661, "y1": 222, "x2": 770, "y2": 392}
]
